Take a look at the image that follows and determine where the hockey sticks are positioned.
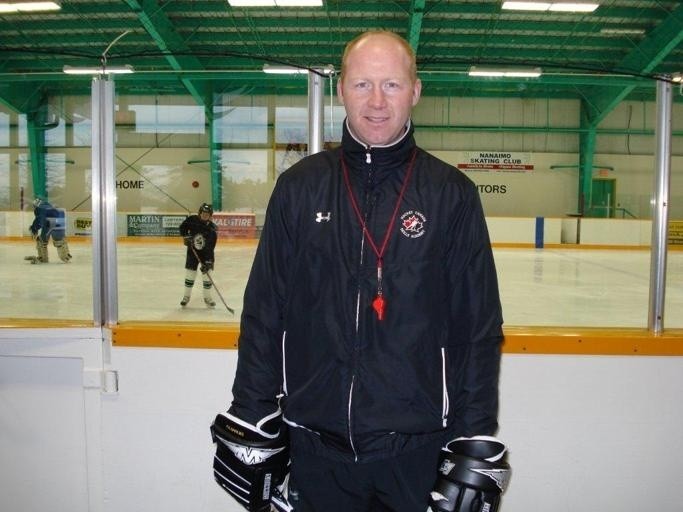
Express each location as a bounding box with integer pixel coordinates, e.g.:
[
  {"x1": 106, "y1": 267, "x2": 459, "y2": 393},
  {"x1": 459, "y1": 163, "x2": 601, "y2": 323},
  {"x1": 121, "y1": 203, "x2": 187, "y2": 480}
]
[{"x1": 191, "y1": 245, "x2": 234, "y2": 315}]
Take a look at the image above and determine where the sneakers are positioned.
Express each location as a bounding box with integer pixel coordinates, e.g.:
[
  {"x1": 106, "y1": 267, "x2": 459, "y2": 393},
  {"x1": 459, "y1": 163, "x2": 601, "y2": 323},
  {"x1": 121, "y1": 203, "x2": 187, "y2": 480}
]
[{"x1": 179, "y1": 295, "x2": 216, "y2": 308}]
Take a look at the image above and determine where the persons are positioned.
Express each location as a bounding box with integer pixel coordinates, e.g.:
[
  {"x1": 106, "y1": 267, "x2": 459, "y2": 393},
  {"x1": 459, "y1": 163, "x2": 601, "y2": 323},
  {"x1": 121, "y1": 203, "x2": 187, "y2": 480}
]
[
  {"x1": 205, "y1": 26, "x2": 512, "y2": 511},
  {"x1": 27, "y1": 197, "x2": 72, "y2": 264},
  {"x1": 177, "y1": 203, "x2": 218, "y2": 310}
]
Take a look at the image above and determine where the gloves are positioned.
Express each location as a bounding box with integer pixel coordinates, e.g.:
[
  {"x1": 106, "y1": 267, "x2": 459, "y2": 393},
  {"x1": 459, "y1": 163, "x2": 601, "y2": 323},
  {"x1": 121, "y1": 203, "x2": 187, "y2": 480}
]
[
  {"x1": 423, "y1": 434, "x2": 511, "y2": 512},
  {"x1": 209, "y1": 405, "x2": 296, "y2": 512}
]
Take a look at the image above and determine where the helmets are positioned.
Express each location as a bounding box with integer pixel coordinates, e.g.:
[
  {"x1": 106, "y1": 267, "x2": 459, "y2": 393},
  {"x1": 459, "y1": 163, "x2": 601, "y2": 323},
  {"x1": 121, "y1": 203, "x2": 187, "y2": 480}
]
[
  {"x1": 198, "y1": 203, "x2": 213, "y2": 216},
  {"x1": 32, "y1": 199, "x2": 43, "y2": 208}
]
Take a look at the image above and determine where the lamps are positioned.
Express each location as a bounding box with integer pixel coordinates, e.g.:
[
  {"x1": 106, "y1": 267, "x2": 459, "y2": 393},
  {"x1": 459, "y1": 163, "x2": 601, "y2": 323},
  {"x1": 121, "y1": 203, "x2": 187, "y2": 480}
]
[
  {"x1": 258, "y1": 61, "x2": 338, "y2": 75},
  {"x1": 61, "y1": 63, "x2": 135, "y2": 76},
  {"x1": 500, "y1": 0, "x2": 599, "y2": 16},
  {"x1": 226, "y1": 1, "x2": 323, "y2": 9},
  {"x1": 468, "y1": 64, "x2": 543, "y2": 78},
  {"x1": 1, "y1": 1, "x2": 63, "y2": 14}
]
